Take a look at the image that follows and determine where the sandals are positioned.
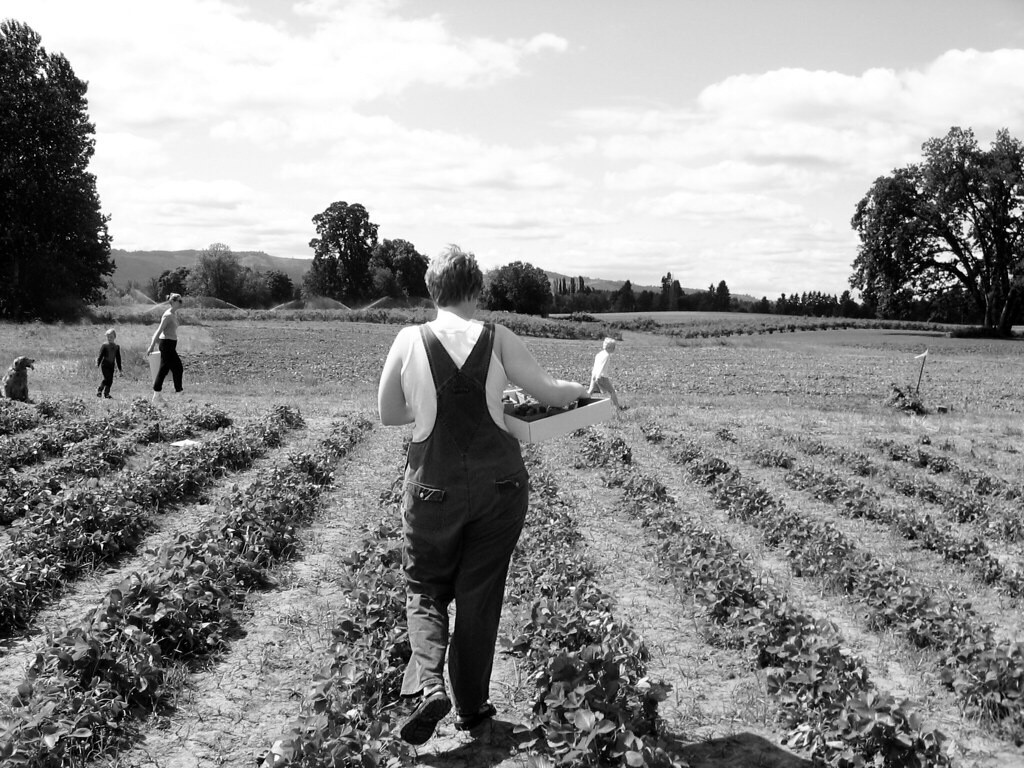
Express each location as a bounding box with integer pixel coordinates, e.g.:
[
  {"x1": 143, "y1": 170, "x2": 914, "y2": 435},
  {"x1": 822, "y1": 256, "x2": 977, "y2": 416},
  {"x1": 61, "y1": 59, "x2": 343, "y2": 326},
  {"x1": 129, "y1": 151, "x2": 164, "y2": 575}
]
[
  {"x1": 399, "y1": 689, "x2": 452, "y2": 746},
  {"x1": 454, "y1": 699, "x2": 497, "y2": 731}
]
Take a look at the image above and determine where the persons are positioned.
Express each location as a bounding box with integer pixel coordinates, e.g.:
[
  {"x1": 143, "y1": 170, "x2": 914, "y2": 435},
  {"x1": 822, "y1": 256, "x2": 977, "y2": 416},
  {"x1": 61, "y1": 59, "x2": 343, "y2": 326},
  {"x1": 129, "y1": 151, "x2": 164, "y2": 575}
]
[
  {"x1": 96, "y1": 329, "x2": 124, "y2": 399},
  {"x1": 378, "y1": 243, "x2": 590, "y2": 745},
  {"x1": 147, "y1": 293, "x2": 186, "y2": 405},
  {"x1": 588, "y1": 338, "x2": 630, "y2": 411}
]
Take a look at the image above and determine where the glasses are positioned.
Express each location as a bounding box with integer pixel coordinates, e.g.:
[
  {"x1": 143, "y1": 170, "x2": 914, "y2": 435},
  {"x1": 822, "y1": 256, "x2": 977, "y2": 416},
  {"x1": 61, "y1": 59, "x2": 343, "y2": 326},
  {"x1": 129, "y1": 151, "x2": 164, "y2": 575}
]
[{"x1": 172, "y1": 299, "x2": 183, "y2": 304}]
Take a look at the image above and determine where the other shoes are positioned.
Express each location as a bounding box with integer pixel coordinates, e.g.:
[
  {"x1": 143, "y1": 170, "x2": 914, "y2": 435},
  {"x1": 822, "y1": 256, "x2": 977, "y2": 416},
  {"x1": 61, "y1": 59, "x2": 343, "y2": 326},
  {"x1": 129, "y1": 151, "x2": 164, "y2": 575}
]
[
  {"x1": 152, "y1": 396, "x2": 167, "y2": 404},
  {"x1": 96, "y1": 392, "x2": 102, "y2": 397},
  {"x1": 104, "y1": 395, "x2": 112, "y2": 399},
  {"x1": 175, "y1": 392, "x2": 191, "y2": 399}
]
